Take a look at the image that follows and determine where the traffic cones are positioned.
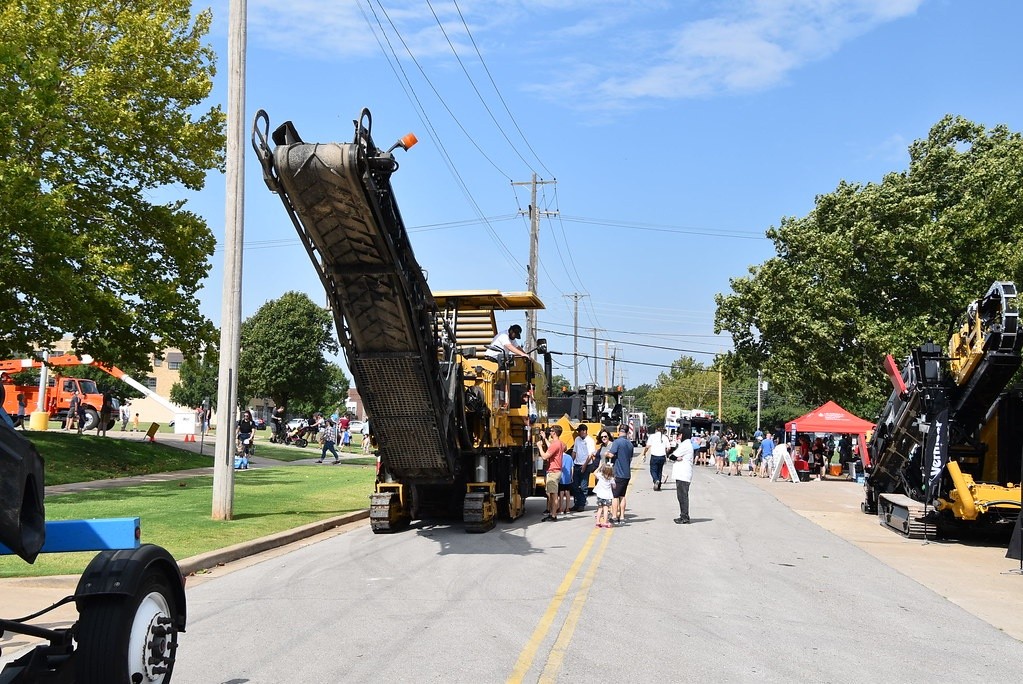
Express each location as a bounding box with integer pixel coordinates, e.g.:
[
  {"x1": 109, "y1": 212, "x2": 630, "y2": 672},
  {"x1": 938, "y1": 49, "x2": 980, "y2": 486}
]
[
  {"x1": 149, "y1": 435, "x2": 155, "y2": 442},
  {"x1": 190, "y1": 434, "x2": 196, "y2": 442},
  {"x1": 184, "y1": 434, "x2": 189, "y2": 442}
]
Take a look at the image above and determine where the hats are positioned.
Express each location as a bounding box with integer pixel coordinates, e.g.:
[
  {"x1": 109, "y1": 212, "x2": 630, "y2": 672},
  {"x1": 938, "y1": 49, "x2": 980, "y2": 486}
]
[
  {"x1": 110, "y1": 386, "x2": 115, "y2": 391},
  {"x1": 756, "y1": 436, "x2": 763, "y2": 441},
  {"x1": 508, "y1": 325, "x2": 522, "y2": 340},
  {"x1": 324, "y1": 419, "x2": 332, "y2": 422},
  {"x1": 575, "y1": 424, "x2": 587, "y2": 431}
]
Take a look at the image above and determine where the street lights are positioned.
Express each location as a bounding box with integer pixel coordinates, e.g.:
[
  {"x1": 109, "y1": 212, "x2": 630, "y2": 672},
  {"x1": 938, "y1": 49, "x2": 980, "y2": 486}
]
[{"x1": 758, "y1": 381, "x2": 768, "y2": 428}]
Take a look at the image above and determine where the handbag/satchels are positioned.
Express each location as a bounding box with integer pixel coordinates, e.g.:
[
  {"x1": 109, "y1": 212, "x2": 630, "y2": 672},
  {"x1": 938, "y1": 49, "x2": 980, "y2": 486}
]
[{"x1": 708, "y1": 454, "x2": 715, "y2": 466}]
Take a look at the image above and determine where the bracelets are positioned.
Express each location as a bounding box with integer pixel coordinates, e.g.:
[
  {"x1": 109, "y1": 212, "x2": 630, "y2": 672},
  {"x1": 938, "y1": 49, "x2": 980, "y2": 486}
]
[{"x1": 74, "y1": 410, "x2": 77, "y2": 412}]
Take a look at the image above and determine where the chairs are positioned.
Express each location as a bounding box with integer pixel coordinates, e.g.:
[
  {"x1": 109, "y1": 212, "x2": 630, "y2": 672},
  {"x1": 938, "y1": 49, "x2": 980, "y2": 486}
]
[{"x1": 809, "y1": 461, "x2": 819, "y2": 478}]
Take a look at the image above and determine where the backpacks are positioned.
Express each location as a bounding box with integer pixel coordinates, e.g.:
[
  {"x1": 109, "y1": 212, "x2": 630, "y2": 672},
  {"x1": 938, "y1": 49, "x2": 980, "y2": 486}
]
[{"x1": 717, "y1": 442, "x2": 724, "y2": 450}]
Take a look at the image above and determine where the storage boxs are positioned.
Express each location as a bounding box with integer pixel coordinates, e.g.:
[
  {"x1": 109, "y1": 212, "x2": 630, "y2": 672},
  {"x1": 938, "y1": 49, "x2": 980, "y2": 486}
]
[{"x1": 829, "y1": 463, "x2": 843, "y2": 476}]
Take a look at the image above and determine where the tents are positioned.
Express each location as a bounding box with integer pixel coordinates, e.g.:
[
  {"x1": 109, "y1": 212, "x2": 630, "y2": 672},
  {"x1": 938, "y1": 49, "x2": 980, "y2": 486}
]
[{"x1": 784, "y1": 401, "x2": 878, "y2": 444}]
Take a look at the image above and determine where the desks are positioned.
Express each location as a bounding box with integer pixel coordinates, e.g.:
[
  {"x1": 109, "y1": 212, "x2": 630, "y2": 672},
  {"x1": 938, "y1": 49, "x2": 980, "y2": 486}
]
[{"x1": 781, "y1": 455, "x2": 809, "y2": 479}]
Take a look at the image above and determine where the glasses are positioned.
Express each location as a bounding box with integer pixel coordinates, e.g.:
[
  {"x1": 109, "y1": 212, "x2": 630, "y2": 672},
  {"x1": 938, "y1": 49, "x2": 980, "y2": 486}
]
[{"x1": 600, "y1": 436, "x2": 607, "y2": 438}]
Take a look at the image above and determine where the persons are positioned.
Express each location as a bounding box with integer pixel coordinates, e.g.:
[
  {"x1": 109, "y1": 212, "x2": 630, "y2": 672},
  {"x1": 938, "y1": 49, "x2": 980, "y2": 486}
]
[
  {"x1": 662, "y1": 427, "x2": 744, "y2": 483},
  {"x1": 120, "y1": 403, "x2": 139, "y2": 432},
  {"x1": 62, "y1": 389, "x2": 88, "y2": 435},
  {"x1": 315, "y1": 419, "x2": 341, "y2": 466},
  {"x1": 484, "y1": 325, "x2": 535, "y2": 364},
  {"x1": 536, "y1": 425, "x2": 574, "y2": 523},
  {"x1": 522, "y1": 390, "x2": 538, "y2": 447},
  {"x1": 644, "y1": 426, "x2": 671, "y2": 491},
  {"x1": 11, "y1": 393, "x2": 26, "y2": 431},
  {"x1": 591, "y1": 424, "x2": 634, "y2": 528},
  {"x1": 304, "y1": 412, "x2": 371, "y2": 453},
  {"x1": 668, "y1": 426, "x2": 694, "y2": 524},
  {"x1": 193, "y1": 399, "x2": 211, "y2": 436},
  {"x1": 749, "y1": 426, "x2": 875, "y2": 481},
  {"x1": 566, "y1": 424, "x2": 596, "y2": 512},
  {"x1": 236, "y1": 406, "x2": 256, "y2": 459},
  {"x1": 269, "y1": 404, "x2": 285, "y2": 444},
  {"x1": 97, "y1": 386, "x2": 115, "y2": 436}
]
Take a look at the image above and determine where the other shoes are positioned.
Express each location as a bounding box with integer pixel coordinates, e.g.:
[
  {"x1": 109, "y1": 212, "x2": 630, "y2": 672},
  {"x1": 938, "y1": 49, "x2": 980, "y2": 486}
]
[
  {"x1": 541, "y1": 515, "x2": 557, "y2": 522},
  {"x1": 543, "y1": 509, "x2": 552, "y2": 515},
  {"x1": 315, "y1": 460, "x2": 322, "y2": 463},
  {"x1": 653, "y1": 480, "x2": 660, "y2": 491},
  {"x1": 273, "y1": 440, "x2": 278, "y2": 443},
  {"x1": 270, "y1": 439, "x2": 273, "y2": 442},
  {"x1": 674, "y1": 516, "x2": 690, "y2": 524},
  {"x1": 601, "y1": 522, "x2": 612, "y2": 528},
  {"x1": 333, "y1": 461, "x2": 341, "y2": 465},
  {"x1": 556, "y1": 508, "x2": 564, "y2": 515},
  {"x1": 565, "y1": 508, "x2": 570, "y2": 514},
  {"x1": 596, "y1": 523, "x2": 602, "y2": 528}
]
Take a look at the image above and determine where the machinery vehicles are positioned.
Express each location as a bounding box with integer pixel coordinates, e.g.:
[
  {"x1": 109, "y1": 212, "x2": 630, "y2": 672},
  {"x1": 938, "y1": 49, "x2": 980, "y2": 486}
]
[
  {"x1": 0, "y1": 380, "x2": 190, "y2": 684},
  {"x1": 250, "y1": 107, "x2": 537, "y2": 534},
  {"x1": 0, "y1": 354, "x2": 178, "y2": 430},
  {"x1": 537, "y1": 338, "x2": 624, "y2": 498},
  {"x1": 861, "y1": 280, "x2": 1022, "y2": 546}
]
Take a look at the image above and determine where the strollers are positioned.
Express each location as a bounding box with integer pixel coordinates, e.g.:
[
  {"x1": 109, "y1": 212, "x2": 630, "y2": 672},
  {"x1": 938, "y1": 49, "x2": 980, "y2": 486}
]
[
  {"x1": 234, "y1": 433, "x2": 253, "y2": 469},
  {"x1": 277, "y1": 418, "x2": 314, "y2": 447}
]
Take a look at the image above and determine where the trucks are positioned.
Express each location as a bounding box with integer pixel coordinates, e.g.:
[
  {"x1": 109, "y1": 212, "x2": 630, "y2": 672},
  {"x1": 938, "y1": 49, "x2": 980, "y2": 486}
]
[
  {"x1": 664, "y1": 407, "x2": 723, "y2": 458},
  {"x1": 625, "y1": 412, "x2": 648, "y2": 447}
]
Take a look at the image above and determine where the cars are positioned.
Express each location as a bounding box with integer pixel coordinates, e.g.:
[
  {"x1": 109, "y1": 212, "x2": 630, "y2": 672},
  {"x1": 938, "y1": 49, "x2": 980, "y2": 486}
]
[
  {"x1": 737, "y1": 435, "x2": 756, "y2": 445},
  {"x1": 254, "y1": 420, "x2": 266, "y2": 430},
  {"x1": 285, "y1": 419, "x2": 309, "y2": 430},
  {"x1": 348, "y1": 421, "x2": 364, "y2": 434}
]
